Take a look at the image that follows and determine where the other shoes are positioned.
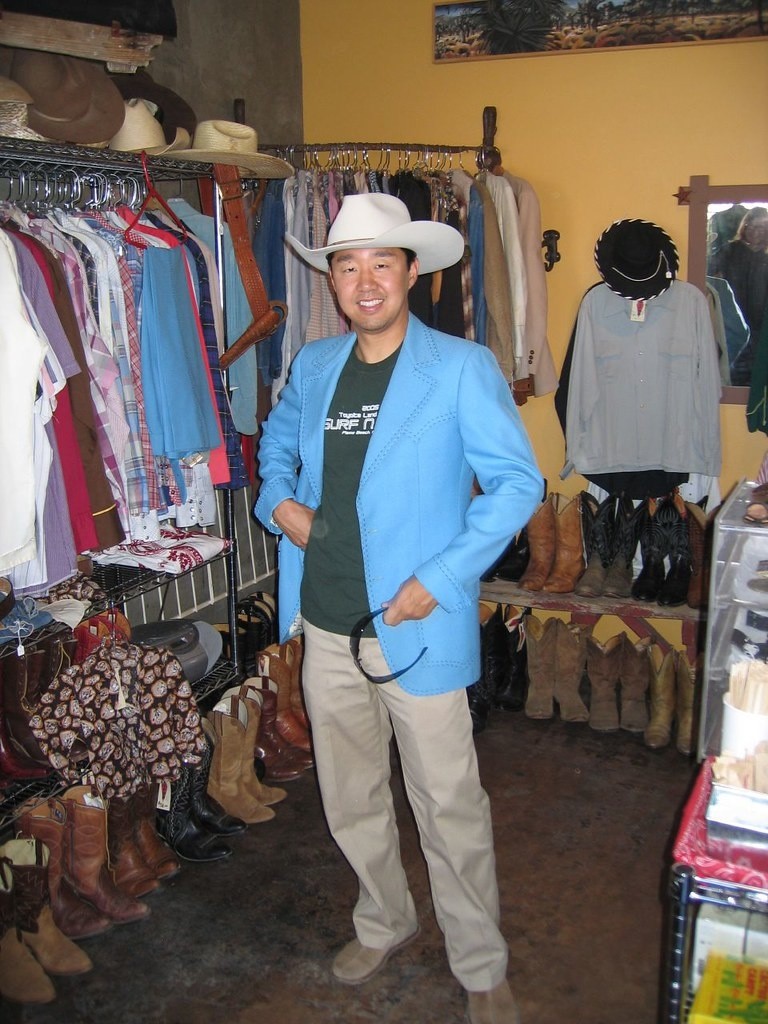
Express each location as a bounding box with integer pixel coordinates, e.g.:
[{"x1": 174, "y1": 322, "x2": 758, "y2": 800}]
[
  {"x1": 730, "y1": 610, "x2": 768, "y2": 665},
  {"x1": 742, "y1": 482, "x2": 768, "y2": 528},
  {"x1": 747, "y1": 561, "x2": 768, "y2": 594},
  {"x1": 467, "y1": 976, "x2": 520, "y2": 1024},
  {"x1": 331, "y1": 920, "x2": 422, "y2": 986}
]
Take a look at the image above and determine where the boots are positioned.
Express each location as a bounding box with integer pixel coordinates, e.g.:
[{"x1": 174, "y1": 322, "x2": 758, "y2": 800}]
[
  {"x1": 478, "y1": 477, "x2": 722, "y2": 609},
  {"x1": 0, "y1": 591, "x2": 318, "y2": 1004},
  {"x1": 464, "y1": 602, "x2": 704, "y2": 758}
]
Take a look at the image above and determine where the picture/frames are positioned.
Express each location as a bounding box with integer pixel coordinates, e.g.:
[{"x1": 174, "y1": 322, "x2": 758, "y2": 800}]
[{"x1": 432, "y1": 0, "x2": 768, "y2": 65}]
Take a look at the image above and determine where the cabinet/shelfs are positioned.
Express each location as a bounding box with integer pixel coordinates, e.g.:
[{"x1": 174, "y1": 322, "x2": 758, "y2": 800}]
[{"x1": 664, "y1": 478, "x2": 767, "y2": 1024}]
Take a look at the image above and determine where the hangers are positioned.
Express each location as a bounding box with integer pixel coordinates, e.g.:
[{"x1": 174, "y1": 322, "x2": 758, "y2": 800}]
[
  {"x1": 260, "y1": 144, "x2": 506, "y2": 187},
  {"x1": 0, "y1": 150, "x2": 188, "y2": 249}
]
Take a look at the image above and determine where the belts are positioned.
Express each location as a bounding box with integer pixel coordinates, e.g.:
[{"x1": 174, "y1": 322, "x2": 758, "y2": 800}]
[{"x1": 0, "y1": 577, "x2": 16, "y2": 621}]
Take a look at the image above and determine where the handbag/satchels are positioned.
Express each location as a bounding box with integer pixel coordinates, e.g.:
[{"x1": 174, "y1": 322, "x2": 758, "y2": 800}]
[{"x1": 82, "y1": 519, "x2": 234, "y2": 575}]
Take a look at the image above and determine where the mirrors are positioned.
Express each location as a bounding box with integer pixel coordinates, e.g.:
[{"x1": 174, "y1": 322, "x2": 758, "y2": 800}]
[{"x1": 673, "y1": 174, "x2": 768, "y2": 406}]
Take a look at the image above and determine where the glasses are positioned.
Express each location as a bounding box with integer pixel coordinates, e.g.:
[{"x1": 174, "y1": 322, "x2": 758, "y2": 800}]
[
  {"x1": 350, "y1": 608, "x2": 430, "y2": 684},
  {"x1": 744, "y1": 223, "x2": 768, "y2": 232}
]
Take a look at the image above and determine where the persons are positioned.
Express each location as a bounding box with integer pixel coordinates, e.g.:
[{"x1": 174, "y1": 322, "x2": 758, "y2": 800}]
[
  {"x1": 720, "y1": 206, "x2": 768, "y2": 376},
  {"x1": 252, "y1": 193, "x2": 547, "y2": 1024}
]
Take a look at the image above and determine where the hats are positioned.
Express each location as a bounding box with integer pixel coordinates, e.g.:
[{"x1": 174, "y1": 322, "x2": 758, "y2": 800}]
[
  {"x1": 284, "y1": 193, "x2": 464, "y2": 275},
  {"x1": 157, "y1": 119, "x2": 295, "y2": 179},
  {"x1": 0, "y1": 46, "x2": 197, "y2": 156},
  {"x1": 593, "y1": 217, "x2": 681, "y2": 301}
]
